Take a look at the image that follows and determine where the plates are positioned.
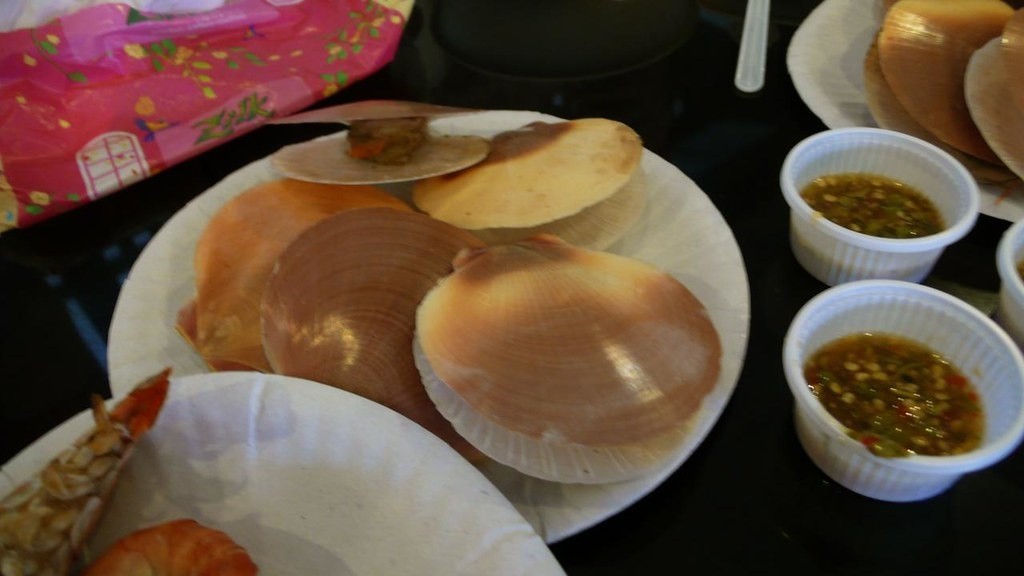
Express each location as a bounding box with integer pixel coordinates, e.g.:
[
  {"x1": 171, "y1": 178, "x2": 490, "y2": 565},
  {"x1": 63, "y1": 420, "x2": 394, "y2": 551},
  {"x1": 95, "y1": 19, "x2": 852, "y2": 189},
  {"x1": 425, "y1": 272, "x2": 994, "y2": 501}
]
[
  {"x1": 0, "y1": 370, "x2": 568, "y2": 576},
  {"x1": 104, "y1": 107, "x2": 751, "y2": 540},
  {"x1": 777, "y1": 0, "x2": 1024, "y2": 223}
]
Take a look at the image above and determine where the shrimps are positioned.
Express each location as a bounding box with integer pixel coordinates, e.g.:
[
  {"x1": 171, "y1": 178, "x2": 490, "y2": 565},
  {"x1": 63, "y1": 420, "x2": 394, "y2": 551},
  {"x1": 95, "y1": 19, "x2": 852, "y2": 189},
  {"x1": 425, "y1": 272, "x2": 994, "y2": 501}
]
[{"x1": 0, "y1": 362, "x2": 264, "y2": 576}]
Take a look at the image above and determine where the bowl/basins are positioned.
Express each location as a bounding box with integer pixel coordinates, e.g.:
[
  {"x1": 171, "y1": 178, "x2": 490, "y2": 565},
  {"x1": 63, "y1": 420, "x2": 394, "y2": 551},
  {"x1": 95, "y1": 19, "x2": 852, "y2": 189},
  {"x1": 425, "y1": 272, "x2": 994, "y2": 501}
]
[
  {"x1": 779, "y1": 279, "x2": 1023, "y2": 501},
  {"x1": 777, "y1": 125, "x2": 980, "y2": 288},
  {"x1": 996, "y1": 216, "x2": 1023, "y2": 351}
]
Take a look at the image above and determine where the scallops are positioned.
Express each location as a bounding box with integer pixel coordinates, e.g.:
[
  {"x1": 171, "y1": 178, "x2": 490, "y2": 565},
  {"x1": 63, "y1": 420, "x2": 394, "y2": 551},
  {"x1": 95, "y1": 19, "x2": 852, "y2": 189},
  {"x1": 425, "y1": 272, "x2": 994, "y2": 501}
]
[
  {"x1": 863, "y1": 0, "x2": 1024, "y2": 193},
  {"x1": 173, "y1": 102, "x2": 723, "y2": 485}
]
